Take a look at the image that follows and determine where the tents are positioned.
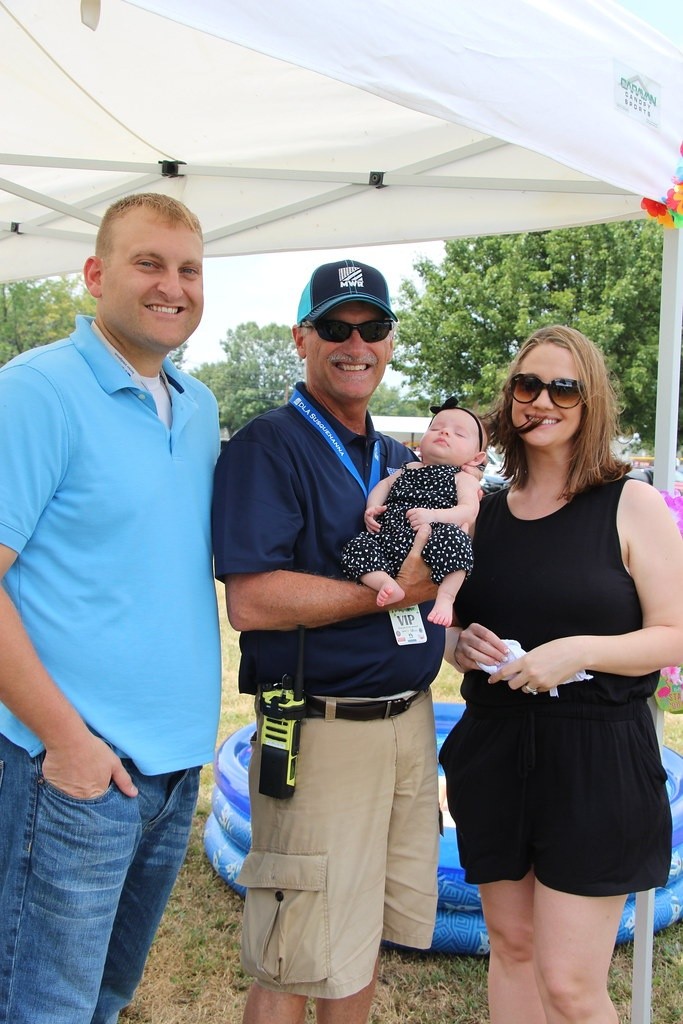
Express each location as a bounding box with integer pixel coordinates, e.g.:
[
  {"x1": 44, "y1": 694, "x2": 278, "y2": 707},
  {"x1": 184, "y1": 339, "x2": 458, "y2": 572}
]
[{"x1": 0, "y1": 1, "x2": 683, "y2": 490}]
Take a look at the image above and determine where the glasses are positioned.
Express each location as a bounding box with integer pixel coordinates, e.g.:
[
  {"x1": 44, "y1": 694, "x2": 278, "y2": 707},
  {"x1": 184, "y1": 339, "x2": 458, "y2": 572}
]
[
  {"x1": 301, "y1": 318, "x2": 393, "y2": 343},
  {"x1": 510, "y1": 373, "x2": 585, "y2": 409}
]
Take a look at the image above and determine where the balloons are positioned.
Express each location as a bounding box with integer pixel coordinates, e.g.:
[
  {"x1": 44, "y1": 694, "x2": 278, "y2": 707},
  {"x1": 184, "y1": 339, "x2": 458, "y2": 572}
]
[
  {"x1": 657, "y1": 490, "x2": 683, "y2": 534},
  {"x1": 642, "y1": 144, "x2": 683, "y2": 230},
  {"x1": 661, "y1": 667, "x2": 683, "y2": 686}
]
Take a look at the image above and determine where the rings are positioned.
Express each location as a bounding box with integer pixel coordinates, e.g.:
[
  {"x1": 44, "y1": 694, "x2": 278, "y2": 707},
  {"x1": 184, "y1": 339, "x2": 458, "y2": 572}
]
[{"x1": 525, "y1": 686, "x2": 538, "y2": 695}]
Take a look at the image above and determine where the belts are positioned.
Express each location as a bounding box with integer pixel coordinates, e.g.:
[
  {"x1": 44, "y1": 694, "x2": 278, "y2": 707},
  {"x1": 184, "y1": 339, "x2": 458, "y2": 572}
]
[{"x1": 303, "y1": 691, "x2": 421, "y2": 720}]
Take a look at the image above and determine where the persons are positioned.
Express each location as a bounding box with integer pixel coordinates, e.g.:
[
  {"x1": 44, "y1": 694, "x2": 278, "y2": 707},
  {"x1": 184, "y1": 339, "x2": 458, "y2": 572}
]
[
  {"x1": 211, "y1": 261, "x2": 446, "y2": 1024},
  {"x1": 341, "y1": 397, "x2": 485, "y2": 628},
  {"x1": 443, "y1": 323, "x2": 682, "y2": 1023},
  {"x1": 0, "y1": 193, "x2": 220, "y2": 1023}
]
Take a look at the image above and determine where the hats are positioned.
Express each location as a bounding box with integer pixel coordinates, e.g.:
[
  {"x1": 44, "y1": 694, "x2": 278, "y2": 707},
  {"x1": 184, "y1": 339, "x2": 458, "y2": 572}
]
[{"x1": 297, "y1": 260, "x2": 398, "y2": 326}]
